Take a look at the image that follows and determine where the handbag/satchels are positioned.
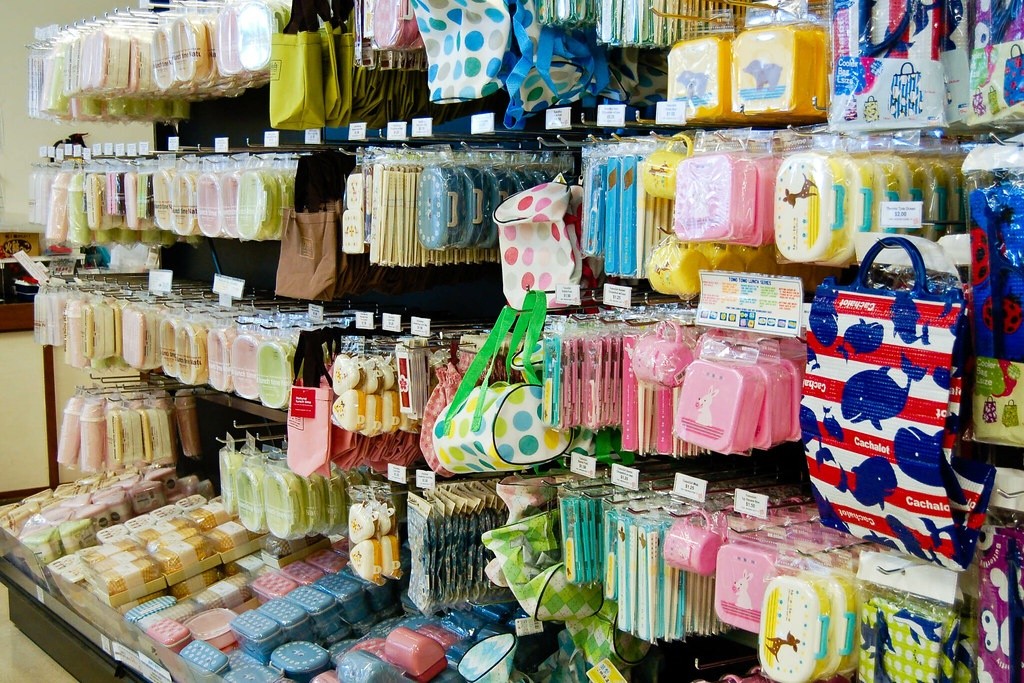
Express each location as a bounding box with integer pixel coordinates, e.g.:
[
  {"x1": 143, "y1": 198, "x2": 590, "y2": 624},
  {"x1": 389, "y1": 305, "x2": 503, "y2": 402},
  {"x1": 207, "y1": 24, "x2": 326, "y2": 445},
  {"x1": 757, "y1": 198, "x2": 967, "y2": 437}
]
[
  {"x1": 975, "y1": 524, "x2": 1024, "y2": 683},
  {"x1": 274, "y1": 148, "x2": 431, "y2": 302},
  {"x1": 481, "y1": 508, "x2": 604, "y2": 622},
  {"x1": 961, "y1": 146, "x2": 1024, "y2": 447},
  {"x1": 799, "y1": 236, "x2": 997, "y2": 571},
  {"x1": 457, "y1": 633, "x2": 533, "y2": 683},
  {"x1": 822, "y1": 0, "x2": 1024, "y2": 134},
  {"x1": 270, "y1": 0, "x2": 671, "y2": 132},
  {"x1": 569, "y1": 594, "x2": 651, "y2": 670},
  {"x1": 539, "y1": 629, "x2": 593, "y2": 683},
  {"x1": 432, "y1": 290, "x2": 574, "y2": 474},
  {"x1": 484, "y1": 474, "x2": 569, "y2": 587},
  {"x1": 492, "y1": 174, "x2": 603, "y2": 310}
]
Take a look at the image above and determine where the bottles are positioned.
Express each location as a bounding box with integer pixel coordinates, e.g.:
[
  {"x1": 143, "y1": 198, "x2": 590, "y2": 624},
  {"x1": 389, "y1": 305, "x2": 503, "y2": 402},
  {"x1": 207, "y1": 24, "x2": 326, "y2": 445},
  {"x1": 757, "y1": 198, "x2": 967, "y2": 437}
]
[
  {"x1": 65, "y1": 299, "x2": 82, "y2": 367},
  {"x1": 56, "y1": 390, "x2": 124, "y2": 472},
  {"x1": 173, "y1": 390, "x2": 201, "y2": 457},
  {"x1": 153, "y1": 391, "x2": 174, "y2": 463}
]
